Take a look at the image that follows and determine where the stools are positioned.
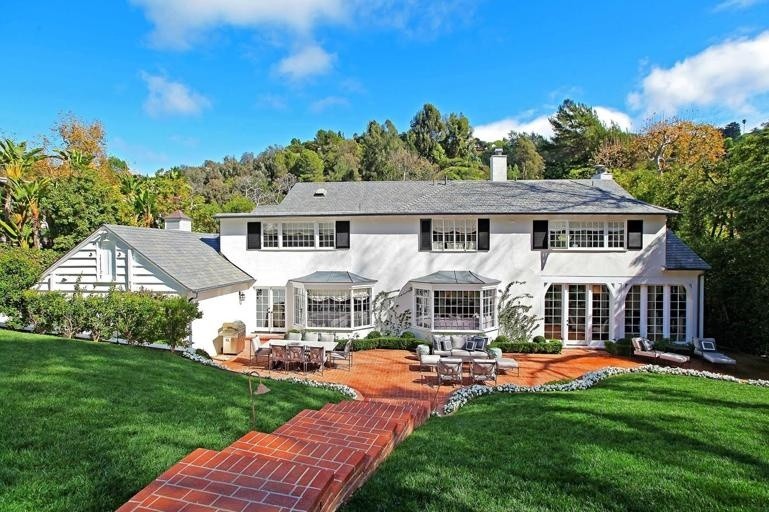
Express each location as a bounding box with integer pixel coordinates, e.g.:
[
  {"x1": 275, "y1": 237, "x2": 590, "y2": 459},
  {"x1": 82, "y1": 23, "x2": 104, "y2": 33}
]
[
  {"x1": 489, "y1": 347, "x2": 520, "y2": 376},
  {"x1": 416, "y1": 344, "x2": 440, "y2": 373}
]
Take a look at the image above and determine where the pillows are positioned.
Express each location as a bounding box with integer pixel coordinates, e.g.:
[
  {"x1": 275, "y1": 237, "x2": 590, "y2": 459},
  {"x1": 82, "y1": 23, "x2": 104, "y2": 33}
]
[
  {"x1": 443, "y1": 340, "x2": 453, "y2": 350},
  {"x1": 642, "y1": 338, "x2": 656, "y2": 350},
  {"x1": 699, "y1": 340, "x2": 715, "y2": 351},
  {"x1": 465, "y1": 337, "x2": 488, "y2": 352}
]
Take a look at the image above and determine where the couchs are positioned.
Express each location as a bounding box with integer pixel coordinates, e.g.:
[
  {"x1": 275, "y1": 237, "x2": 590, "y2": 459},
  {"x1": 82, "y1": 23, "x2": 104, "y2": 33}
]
[{"x1": 432, "y1": 334, "x2": 489, "y2": 359}]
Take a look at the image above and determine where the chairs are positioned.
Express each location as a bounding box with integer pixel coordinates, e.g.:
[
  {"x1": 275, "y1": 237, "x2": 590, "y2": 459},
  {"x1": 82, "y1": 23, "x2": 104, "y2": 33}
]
[
  {"x1": 249, "y1": 332, "x2": 354, "y2": 377},
  {"x1": 436, "y1": 355, "x2": 497, "y2": 385},
  {"x1": 630, "y1": 335, "x2": 691, "y2": 366},
  {"x1": 691, "y1": 336, "x2": 736, "y2": 369}
]
